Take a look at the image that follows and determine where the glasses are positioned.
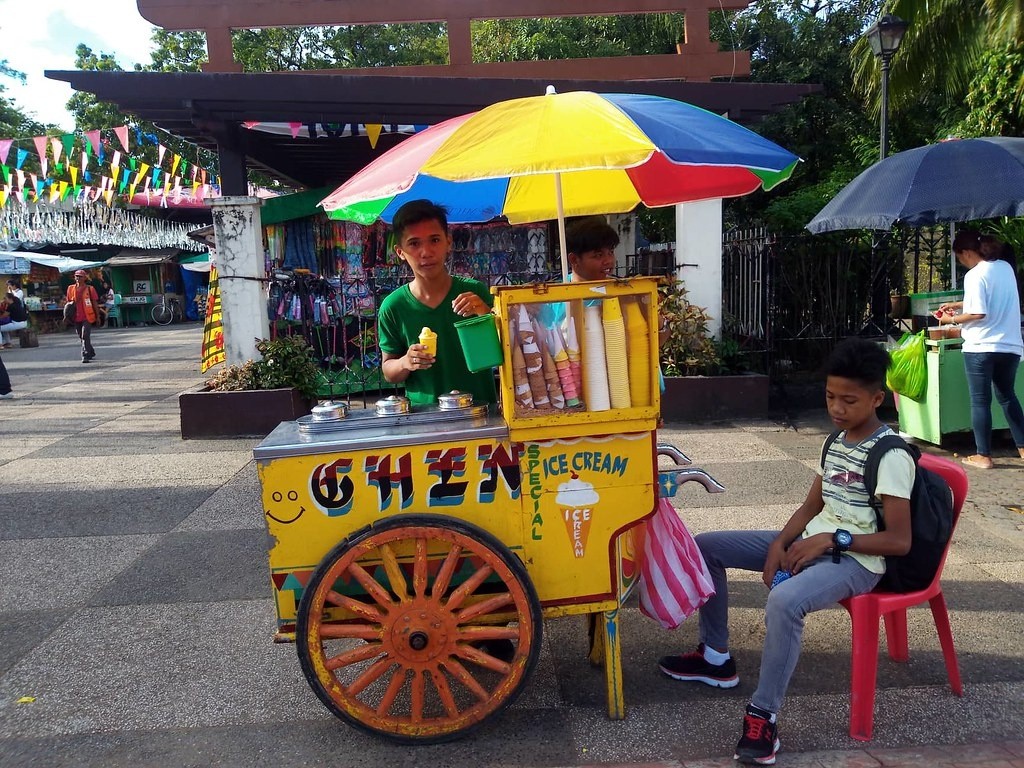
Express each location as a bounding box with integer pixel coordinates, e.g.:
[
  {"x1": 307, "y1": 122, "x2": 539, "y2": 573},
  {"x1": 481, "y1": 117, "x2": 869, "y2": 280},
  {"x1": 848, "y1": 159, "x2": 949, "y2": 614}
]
[{"x1": 76, "y1": 275, "x2": 83, "y2": 278}]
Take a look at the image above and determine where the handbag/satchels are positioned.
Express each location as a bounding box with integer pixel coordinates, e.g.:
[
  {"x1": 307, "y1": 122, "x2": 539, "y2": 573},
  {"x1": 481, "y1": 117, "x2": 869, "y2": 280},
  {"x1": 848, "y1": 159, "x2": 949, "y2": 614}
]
[
  {"x1": 636, "y1": 500, "x2": 716, "y2": 631},
  {"x1": 883, "y1": 330, "x2": 926, "y2": 402}
]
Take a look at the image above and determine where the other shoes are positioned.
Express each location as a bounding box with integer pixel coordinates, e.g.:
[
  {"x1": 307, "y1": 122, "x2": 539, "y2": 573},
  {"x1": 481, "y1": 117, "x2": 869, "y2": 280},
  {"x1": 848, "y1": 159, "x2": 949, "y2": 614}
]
[
  {"x1": 0, "y1": 344, "x2": 4, "y2": 350},
  {"x1": 100, "y1": 324, "x2": 108, "y2": 329},
  {"x1": 0, "y1": 391, "x2": 14, "y2": 400},
  {"x1": 105, "y1": 313, "x2": 109, "y2": 320},
  {"x1": 4, "y1": 343, "x2": 12, "y2": 348},
  {"x1": 81, "y1": 357, "x2": 90, "y2": 363},
  {"x1": 89, "y1": 352, "x2": 96, "y2": 359}
]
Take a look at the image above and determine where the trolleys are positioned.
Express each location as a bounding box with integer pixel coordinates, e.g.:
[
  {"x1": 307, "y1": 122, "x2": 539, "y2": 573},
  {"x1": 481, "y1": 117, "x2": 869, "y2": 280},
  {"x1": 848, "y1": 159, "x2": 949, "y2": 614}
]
[{"x1": 253, "y1": 276, "x2": 727, "y2": 747}]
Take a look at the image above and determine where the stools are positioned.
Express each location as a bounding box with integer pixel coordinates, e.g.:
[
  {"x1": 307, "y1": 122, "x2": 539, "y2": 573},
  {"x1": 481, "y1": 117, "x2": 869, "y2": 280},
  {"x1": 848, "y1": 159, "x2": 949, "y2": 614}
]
[{"x1": 19, "y1": 327, "x2": 38, "y2": 347}]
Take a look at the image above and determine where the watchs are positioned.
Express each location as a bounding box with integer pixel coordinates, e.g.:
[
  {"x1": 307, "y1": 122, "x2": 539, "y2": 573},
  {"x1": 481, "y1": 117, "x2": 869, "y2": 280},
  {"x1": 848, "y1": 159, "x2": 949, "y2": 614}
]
[{"x1": 832, "y1": 528, "x2": 853, "y2": 563}]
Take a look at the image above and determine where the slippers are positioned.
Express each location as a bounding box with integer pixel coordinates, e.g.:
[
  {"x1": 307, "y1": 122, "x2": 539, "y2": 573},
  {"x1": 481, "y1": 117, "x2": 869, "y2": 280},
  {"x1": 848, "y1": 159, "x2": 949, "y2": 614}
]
[{"x1": 961, "y1": 454, "x2": 993, "y2": 469}]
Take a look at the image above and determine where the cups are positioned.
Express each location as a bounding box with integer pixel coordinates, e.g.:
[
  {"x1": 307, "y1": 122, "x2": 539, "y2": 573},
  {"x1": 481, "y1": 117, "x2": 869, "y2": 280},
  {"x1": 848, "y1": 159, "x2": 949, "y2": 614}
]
[
  {"x1": 419, "y1": 331, "x2": 437, "y2": 357},
  {"x1": 583, "y1": 296, "x2": 650, "y2": 412}
]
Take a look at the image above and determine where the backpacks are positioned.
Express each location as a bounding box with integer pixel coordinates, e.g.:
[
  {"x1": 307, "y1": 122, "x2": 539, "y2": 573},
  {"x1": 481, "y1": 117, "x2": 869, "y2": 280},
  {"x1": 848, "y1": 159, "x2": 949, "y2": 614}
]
[{"x1": 821, "y1": 429, "x2": 955, "y2": 595}]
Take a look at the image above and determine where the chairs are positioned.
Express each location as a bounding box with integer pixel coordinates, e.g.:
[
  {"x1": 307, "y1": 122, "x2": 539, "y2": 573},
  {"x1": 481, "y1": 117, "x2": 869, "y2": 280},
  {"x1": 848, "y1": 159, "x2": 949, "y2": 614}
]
[
  {"x1": 106, "y1": 294, "x2": 124, "y2": 327},
  {"x1": 817, "y1": 452, "x2": 967, "y2": 741}
]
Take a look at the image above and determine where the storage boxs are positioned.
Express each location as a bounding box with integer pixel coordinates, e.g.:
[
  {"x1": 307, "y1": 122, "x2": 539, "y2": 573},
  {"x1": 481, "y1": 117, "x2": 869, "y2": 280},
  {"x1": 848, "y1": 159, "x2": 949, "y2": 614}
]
[
  {"x1": 911, "y1": 290, "x2": 964, "y2": 317},
  {"x1": 912, "y1": 315, "x2": 947, "y2": 338}
]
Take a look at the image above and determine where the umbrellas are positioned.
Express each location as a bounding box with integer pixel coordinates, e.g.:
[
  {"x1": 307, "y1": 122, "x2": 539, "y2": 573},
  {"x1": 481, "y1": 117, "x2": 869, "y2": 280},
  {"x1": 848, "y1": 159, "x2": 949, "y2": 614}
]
[
  {"x1": 805, "y1": 135, "x2": 1024, "y2": 291},
  {"x1": 314, "y1": 85, "x2": 802, "y2": 285}
]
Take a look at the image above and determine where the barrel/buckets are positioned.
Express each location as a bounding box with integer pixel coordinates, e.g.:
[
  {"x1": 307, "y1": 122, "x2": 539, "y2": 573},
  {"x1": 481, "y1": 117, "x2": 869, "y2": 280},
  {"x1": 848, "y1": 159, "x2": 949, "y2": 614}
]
[{"x1": 453, "y1": 312, "x2": 503, "y2": 373}]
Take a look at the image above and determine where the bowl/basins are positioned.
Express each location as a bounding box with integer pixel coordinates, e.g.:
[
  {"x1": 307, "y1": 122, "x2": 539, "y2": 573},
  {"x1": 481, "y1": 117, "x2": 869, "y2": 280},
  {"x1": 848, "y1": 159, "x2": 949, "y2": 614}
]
[{"x1": 927, "y1": 324, "x2": 962, "y2": 350}]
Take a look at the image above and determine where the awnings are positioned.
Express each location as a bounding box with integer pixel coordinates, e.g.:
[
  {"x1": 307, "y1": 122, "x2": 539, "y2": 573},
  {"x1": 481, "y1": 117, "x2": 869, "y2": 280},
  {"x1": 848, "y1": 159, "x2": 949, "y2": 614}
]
[{"x1": 32, "y1": 256, "x2": 109, "y2": 273}]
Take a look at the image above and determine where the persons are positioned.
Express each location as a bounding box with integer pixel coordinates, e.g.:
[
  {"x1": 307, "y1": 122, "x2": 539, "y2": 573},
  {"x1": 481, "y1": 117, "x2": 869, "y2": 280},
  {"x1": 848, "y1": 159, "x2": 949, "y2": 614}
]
[
  {"x1": 976, "y1": 235, "x2": 1018, "y2": 280},
  {"x1": 0, "y1": 356, "x2": 14, "y2": 400},
  {"x1": 377, "y1": 200, "x2": 498, "y2": 412},
  {"x1": 0, "y1": 279, "x2": 28, "y2": 350},
  {"x1": 97, "y1": 280, "x2": 117, "y2": 328},
  {"x1": 933, "y1": 232, "x2": 1024, "y2": 468},
  {"x1": 659, "y1": 338, "x2": 916, "y2": 765},
  {"x1": 62, "y1": 270, "x2": 101, "y2": 364},
  {"x1": 536, "y1": 223, "x2": 670, "y2": 398}
]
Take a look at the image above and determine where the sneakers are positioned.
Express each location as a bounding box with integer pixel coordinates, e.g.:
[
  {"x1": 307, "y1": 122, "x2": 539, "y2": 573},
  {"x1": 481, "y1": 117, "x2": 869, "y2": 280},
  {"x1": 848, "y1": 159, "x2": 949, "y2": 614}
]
[
  {"x1": 658, "y1": 642, "x2": 739, "y2": 688},
  {"x1": 733, "y1": 704, "x2": 780, "y2": 765}
]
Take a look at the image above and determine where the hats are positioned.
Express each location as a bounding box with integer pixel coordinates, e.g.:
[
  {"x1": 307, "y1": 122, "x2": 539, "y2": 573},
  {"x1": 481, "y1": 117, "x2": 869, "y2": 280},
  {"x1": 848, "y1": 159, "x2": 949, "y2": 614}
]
[{"x1": 74, "y1": 269, "x2": 86, "y2": 276}]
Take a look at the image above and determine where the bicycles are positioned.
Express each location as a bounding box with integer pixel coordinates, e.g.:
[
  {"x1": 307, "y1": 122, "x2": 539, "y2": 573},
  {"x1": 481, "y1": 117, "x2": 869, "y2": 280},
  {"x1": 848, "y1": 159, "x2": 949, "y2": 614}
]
[{"x1": 150, "y1": 286, "x2": 183, "y2": 326}]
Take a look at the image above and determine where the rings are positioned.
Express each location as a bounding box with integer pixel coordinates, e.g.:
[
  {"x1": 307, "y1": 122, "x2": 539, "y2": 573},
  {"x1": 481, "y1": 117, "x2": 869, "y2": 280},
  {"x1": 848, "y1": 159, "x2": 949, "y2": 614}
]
[{"x1": 413, "y1": 358, "x2": 416, "y2": 363}]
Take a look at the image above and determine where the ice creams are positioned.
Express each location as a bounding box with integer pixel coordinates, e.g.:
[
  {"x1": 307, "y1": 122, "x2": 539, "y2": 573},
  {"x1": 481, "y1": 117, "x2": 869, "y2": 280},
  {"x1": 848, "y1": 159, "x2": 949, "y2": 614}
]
[{"x1": 419, "y1": 327, "x2": 438, "y2": 358}]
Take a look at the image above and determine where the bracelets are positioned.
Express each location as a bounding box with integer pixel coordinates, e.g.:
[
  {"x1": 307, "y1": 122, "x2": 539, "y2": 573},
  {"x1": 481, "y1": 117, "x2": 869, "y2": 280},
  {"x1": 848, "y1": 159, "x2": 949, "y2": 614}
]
[{"x1": 952, "y1": 316, "x2": 958, "y2": 325}]
